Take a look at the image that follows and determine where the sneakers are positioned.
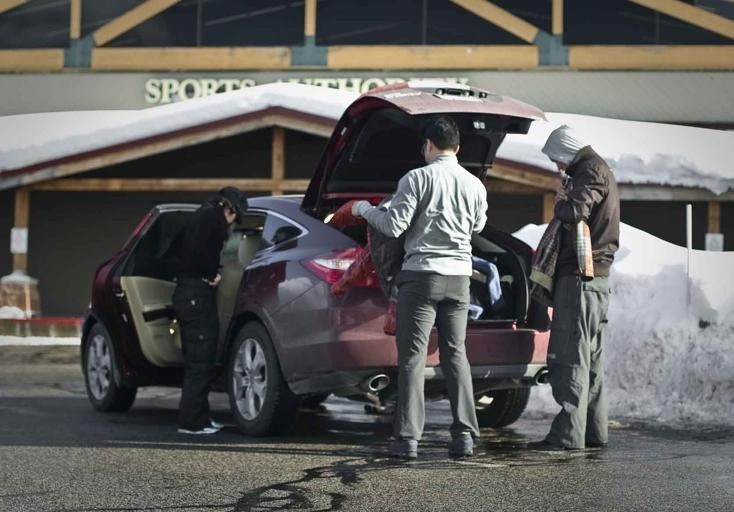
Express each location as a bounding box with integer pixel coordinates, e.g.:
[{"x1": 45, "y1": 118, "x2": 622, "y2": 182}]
[
  {"x1": 447, "y1": 429, "x2": 474, "y2": 457},
  {"x1": 585, "y1": 442, "x2": 608, "y2": 447},
  {"x1": 387, "y1": 438, "x2": 419, "y2": 458},
  {"x1": 209, "y1": 417, "x2": 225, "y2": 428},
  {"x1": 526, "y1": 440, "x2": 572, "y2": 450},
  {"x1": 177, "y1": 427, "x2": 220, "y2": 435}
]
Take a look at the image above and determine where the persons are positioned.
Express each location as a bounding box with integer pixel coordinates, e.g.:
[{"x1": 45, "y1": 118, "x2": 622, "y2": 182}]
[
  {"x1": 165, "y1": 186, "x2": 251, "y2": 435},
  {"x1": 350, "y1": 116, "x2": 489, "y2": 459},
  {"x1": 525, "y1": 123, "x2": 622, "y2": 449}
]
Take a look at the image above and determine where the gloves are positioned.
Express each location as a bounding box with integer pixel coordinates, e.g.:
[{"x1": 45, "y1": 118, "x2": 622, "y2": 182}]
[{"x1": 351, "y1": 199, "x2": 373, "y2": 218}]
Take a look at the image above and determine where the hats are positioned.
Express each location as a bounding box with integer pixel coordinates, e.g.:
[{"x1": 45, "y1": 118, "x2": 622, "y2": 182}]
[{"x1": 208, "y1": 186, "x2": 248, "y2": 224}]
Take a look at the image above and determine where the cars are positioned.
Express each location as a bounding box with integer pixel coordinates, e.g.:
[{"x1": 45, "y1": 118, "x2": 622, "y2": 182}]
[{"x1": 77, "y1": 84, "x2": 559, "y2": 440}]
